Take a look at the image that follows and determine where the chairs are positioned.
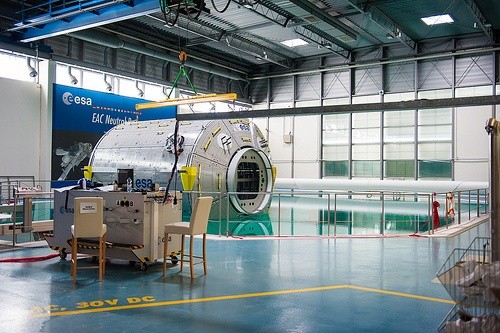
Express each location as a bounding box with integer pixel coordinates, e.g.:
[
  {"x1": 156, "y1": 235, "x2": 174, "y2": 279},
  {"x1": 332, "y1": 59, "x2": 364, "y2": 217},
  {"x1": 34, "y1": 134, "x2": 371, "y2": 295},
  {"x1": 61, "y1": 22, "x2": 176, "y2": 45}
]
[
  {"x1": 70, "y1": 197, "x2": 107, "y2": 280},
  {"x1": 162, "y1": 196, "x2": 214, "y2": 280}
]
